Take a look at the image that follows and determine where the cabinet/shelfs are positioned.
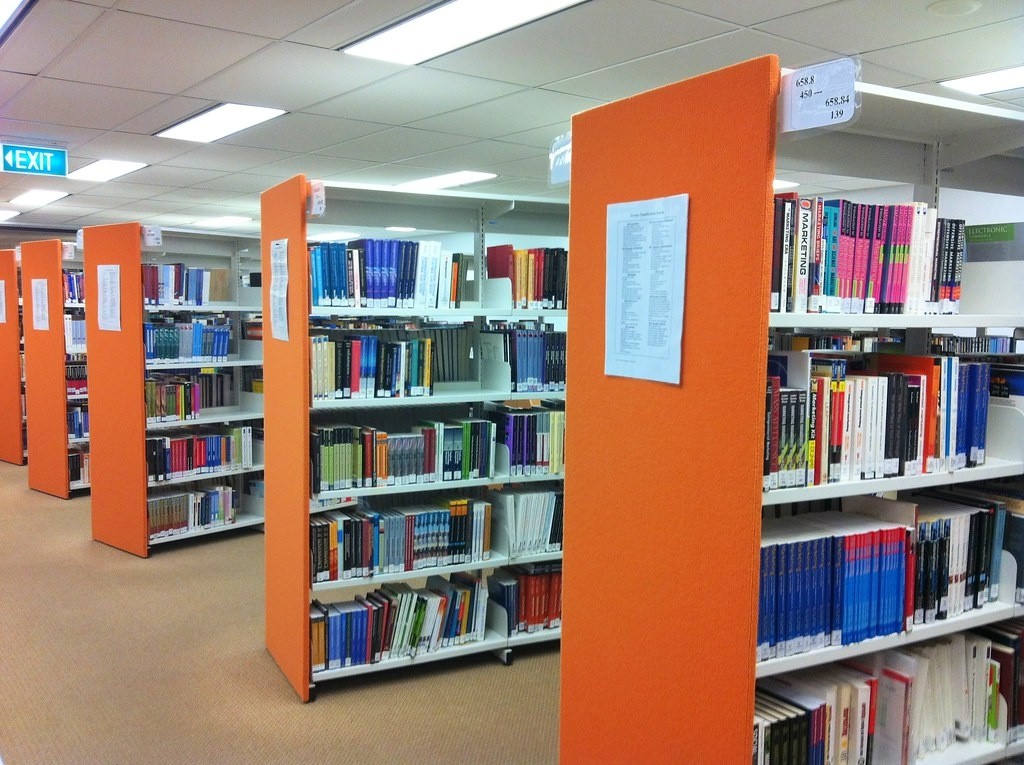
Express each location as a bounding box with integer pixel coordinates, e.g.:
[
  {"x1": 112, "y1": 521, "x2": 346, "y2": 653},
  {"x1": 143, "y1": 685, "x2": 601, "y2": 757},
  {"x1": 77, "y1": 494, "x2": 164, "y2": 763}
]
[
  {"x1": 0, "y1": 248, "x2": 27, "y2": 466},
  {"x1": 260, "y1": 174, "x2": 568, "y2": 704},
  {"x1": 83, "y1": 223, "x2": 265, "y2": 558},
  {"x1": 20, "y1": 239, "x2": 90, "y2": 500},
  {"x1": 558, "y1": 53, "x2": 1024, "y2": 764}
]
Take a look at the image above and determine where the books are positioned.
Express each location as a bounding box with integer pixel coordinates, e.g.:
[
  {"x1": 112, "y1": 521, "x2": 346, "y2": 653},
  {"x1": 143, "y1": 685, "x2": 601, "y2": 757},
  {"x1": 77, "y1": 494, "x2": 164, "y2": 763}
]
[
  {"x1": 753, "y1": 193, "x2": 1024, "y2": 765},
  {"x1": 16, "y1": 268, "x2": 90, "y2": 489},
  {"x1": 140, "y1": 263, "x2": 266, "y2": 542},
  {"x1": 305, "y1": 236, "x2": 570, "y2": 672}
]
